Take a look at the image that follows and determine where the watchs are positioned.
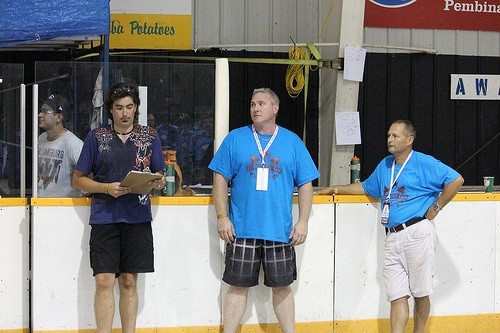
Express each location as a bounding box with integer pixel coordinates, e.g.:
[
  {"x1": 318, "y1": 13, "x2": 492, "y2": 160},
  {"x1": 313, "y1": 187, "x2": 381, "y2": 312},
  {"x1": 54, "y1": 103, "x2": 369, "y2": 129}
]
[{"x1": 433, "y1": 202, "x2": 442, "y2": 212}]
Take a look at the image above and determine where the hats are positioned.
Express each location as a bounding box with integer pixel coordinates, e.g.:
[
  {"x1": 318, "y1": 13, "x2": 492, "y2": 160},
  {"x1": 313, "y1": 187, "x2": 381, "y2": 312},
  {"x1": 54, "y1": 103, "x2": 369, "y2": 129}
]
[{"x1": 39, "y1": 94, "x2": 71, "y2": 121}]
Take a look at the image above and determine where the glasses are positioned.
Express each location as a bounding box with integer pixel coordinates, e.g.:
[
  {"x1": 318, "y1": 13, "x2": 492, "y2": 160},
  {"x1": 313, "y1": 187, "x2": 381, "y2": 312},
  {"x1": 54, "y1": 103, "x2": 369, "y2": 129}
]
[
  {"x1": 40, "y1": 109, "x2": 60, "y2": 115},
  {"x1": 113, "y1": 86, "x2": 138, "y2": 95}
]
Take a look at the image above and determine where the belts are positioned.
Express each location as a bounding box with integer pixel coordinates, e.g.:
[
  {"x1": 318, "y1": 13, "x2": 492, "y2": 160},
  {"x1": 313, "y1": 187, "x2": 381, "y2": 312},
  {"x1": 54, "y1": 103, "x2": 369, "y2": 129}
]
[{"x1": 386, "y1": 217, "x2": 426, "y2": 235}]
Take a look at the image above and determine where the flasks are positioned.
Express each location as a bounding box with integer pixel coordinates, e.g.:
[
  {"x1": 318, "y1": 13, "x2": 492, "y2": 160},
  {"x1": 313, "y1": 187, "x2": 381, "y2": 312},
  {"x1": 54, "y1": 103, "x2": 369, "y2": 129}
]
[{"x1": 162, "y1": 165, "x2": 175, "y2": 197}]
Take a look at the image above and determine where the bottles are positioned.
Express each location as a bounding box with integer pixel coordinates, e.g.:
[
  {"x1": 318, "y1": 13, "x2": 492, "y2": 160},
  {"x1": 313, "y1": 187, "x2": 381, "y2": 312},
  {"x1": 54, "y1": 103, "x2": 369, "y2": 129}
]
[{"x1": 351, "y1": 156, "x2": 360, "y2": 183}]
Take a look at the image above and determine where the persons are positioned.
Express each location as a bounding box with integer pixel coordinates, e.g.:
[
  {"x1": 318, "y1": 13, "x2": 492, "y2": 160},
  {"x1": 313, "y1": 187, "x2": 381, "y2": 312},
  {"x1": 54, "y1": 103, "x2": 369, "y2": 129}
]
[
  {"x1": 38, "y1": 92, "x2": 84, "y2": 198},
  {"x1": 67, "y1": 83, "x2": 166, "y2": 333},
  {"x1": 149, "y1": 107, "x2": 213, "y2": 188},
  {"x1": 313, "y1": 119, "x2": 464, "y2": 333},
  {"x1": 207, "y1": 88, "x2": 322, "y2": 333}
]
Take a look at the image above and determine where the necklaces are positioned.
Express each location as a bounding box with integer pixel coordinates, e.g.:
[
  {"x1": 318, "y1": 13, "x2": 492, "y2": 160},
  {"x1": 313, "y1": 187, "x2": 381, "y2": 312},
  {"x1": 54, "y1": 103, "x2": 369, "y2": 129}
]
[{"x1": 113, "y1": 128, "x2": 135, "y2": 136}]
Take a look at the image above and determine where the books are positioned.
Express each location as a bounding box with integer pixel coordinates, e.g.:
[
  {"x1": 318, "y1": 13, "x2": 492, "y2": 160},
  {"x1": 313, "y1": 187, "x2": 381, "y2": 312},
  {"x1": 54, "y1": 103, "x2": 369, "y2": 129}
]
[{"x1": 118, "y1": 170, "x2": 163, "y2": 195}]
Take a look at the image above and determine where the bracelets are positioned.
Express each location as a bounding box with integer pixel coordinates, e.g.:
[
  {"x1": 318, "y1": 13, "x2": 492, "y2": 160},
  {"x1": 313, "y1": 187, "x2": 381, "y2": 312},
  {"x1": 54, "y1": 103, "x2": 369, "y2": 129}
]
[{"x1": 216, "y1": 213, "x2": 228, "y2": 219}]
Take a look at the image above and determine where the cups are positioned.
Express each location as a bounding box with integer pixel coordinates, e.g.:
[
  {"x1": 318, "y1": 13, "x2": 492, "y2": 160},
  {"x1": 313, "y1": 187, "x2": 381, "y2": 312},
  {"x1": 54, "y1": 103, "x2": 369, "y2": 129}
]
[{"x1": 483, "y1": 177, "x2": 494, "y2": 194}]
